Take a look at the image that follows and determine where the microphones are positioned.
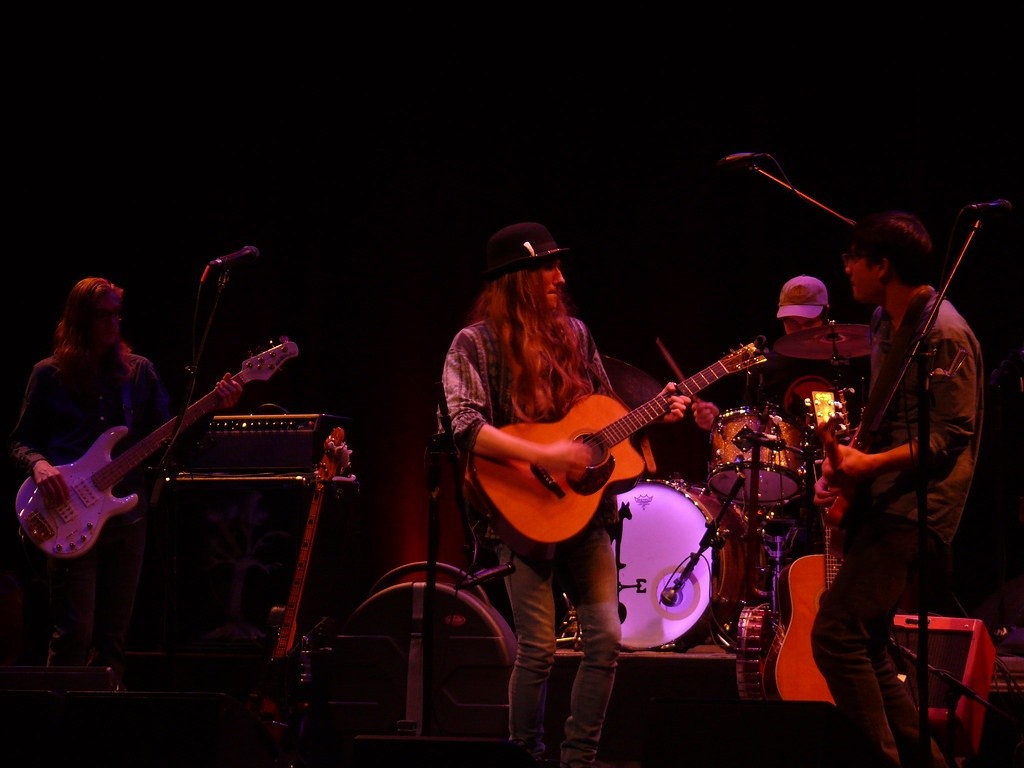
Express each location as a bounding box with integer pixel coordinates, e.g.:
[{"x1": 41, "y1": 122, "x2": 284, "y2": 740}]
[
  {"x1": 209, "y1": 247, "x2": 261, "y2": 271},
  {"x1": 715, "y1": 152, "x2": 770, "y2": 172},
  {"x1": 745, "y1": 339, "x2": 764, "y2": 405},
  {"x1": 659, "y1": 588, "x2": 679, "y2": 606},
  {"x1": 963, "y1": 198, "x2": 1012, "y2": 216},
  {"x1": 455, "y1": 562, "x2": 517, "y2": 591}
]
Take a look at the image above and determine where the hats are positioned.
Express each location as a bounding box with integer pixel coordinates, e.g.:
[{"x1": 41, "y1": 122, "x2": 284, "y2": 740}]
[
  {"x1": 776, "y1": 274, "x2": 829, "y2": 319},
  {"x1": 476, "y1": 221, "x2": 571, "y2": 275}
]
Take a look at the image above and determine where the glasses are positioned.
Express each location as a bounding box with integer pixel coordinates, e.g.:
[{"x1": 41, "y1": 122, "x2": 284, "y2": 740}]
[
  {"x1": 90, "y1": 308, "x2": 125, "y2": 321},
  {"x1": 839, "y1": 247, "x2": 882, "y2": 267}
]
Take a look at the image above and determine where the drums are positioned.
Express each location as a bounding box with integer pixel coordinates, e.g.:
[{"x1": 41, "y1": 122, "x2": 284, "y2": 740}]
[
  {"x1": 706, "y1": 404, "x2": 807, "y2": 508},
  {"x1": 612, "y1": 476, "x2": 748, "y2": 652}
]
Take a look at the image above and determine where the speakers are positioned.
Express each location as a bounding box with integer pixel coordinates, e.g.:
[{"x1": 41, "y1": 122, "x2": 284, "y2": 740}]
[
  {"x1": 164, "y1": 472, "x2": 361, "y2": 656},
  {"x1": 349, "y1": 734, "x2": 547, "y2": 768},
  {"x1": 889, "y1": 613, "x2": 996, "y2": 757},
  {"x1": 0, "y1": 686, "x2": 291, "y2": 768},
  {"x1": 641, "y1": 697, "x2": 901, "y2": 768}
]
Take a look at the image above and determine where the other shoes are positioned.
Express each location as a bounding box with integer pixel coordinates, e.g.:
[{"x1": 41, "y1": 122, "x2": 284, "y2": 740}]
[
  {"x1": 37, "y1": 666, "x2": 79, "y2": 694},
  {"x1": 79, "y1": 665, "x2": 125, "y2": 692}
]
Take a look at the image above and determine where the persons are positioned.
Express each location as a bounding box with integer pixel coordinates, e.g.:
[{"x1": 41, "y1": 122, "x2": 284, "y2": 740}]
[
  {"x1": 438, "y1": 222, "x2": 691, "y2": 768},
  {"x1": 13, "y1": 276, "x2": 242, "y2": 670},
  {"x1": 689, "y1": 274, "x2": 852, "y2": 551},
  {"x1": 812, "y1": 212, "x2": 984, "y2": 768}
]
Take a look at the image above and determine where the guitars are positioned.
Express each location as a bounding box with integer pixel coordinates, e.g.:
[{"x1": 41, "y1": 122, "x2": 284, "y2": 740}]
[
  {"x1": 215, "y1": 424, "x2": 355, "y2": 768},
  {"x1": 822, "y1": 402, "x2": 860, "y2": 562},
  {"x1": 762, "y1": 389, "x2": 848, "y2": 708},
  {"x1": 464, "y1": 338, "x2": 770, "y2": 558},
  {"x1": 14, "y1": 332, "x2": 303, "y2": 559}
]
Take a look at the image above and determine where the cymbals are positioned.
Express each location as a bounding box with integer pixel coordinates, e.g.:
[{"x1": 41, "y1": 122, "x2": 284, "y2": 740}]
[{"x1": 773, "y1": 323, "x2": 876, "y2": 361}]
